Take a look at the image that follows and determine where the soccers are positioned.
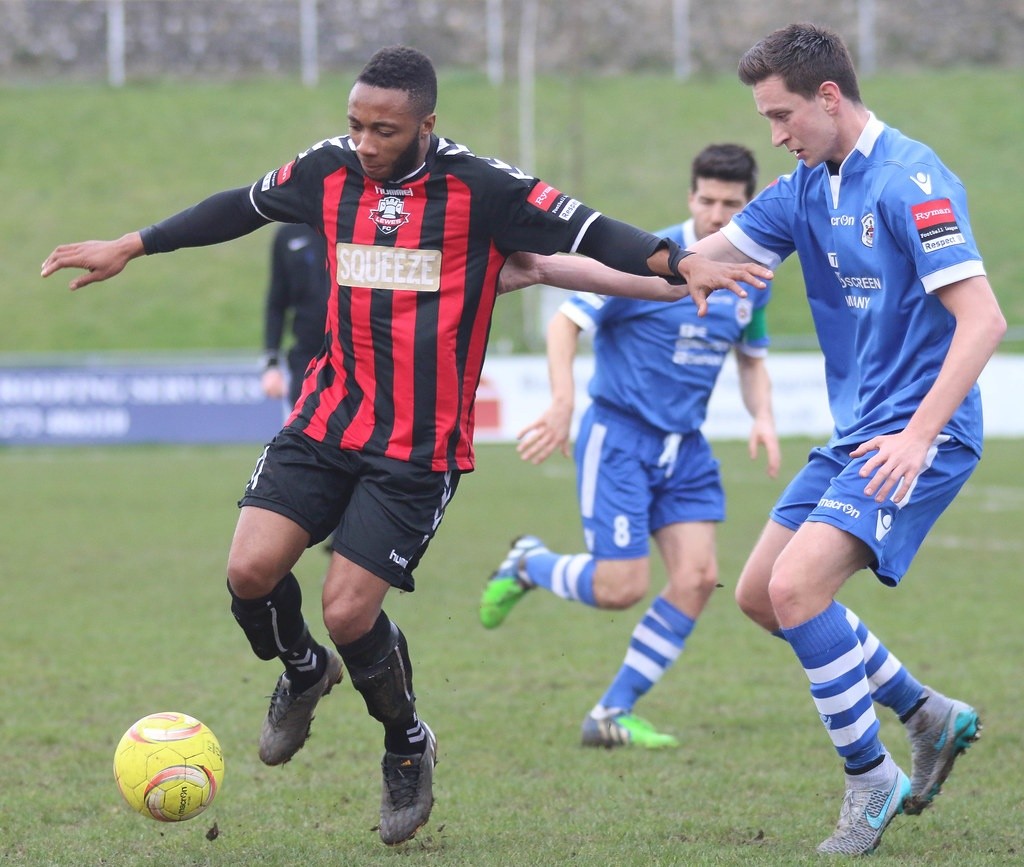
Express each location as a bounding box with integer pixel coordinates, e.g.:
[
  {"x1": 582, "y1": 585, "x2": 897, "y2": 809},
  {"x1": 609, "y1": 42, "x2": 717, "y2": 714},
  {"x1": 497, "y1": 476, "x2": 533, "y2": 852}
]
[{"x1": 113, "y1": 711, "x2": 225, "y2": 822}]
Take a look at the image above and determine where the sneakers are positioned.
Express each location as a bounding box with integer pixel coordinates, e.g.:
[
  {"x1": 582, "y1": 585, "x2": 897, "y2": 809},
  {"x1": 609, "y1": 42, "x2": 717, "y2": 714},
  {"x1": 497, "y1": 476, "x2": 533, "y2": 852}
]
[
  {"x1": 479, "y1": 534, "x2": 546, "y2": 629},
  {"x1": 906, "y1": 699, "x2": 983, "y2": 815},
  {"x1": 257, "y1": 645, "x2": 344, "y2": 766},
  {"x1": 582, "y1": 711, "x2": 680, "y2": 749},
  {"x1": 817, "y1": 767, "x2": 910, "y2": 856},
  {"x1": 379, "y1": 720, "x2": 437, "y2": 846}
]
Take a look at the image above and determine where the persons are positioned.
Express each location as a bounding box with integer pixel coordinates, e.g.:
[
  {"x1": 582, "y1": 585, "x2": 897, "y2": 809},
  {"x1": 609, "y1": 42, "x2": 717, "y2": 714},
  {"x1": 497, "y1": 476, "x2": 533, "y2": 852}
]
[
  {"x1": 495, "y1": 24, "x2": 1006, "y2": 857},
  {"x1": 479, "y1": 145, "x2": 780, "y2": 751},
  {"x1": 261, "y1": 223, "x2": 326, "y2": 412},
  {"x1": 40, "y1": 48, "x2": 774, "y2": 845}
]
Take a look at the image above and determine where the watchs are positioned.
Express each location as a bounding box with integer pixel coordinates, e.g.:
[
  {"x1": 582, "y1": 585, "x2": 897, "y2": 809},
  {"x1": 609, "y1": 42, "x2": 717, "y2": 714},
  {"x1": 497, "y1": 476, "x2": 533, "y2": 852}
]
[{"x1": 260, "y1": 356, "x2": 279, "y2": 368}]
